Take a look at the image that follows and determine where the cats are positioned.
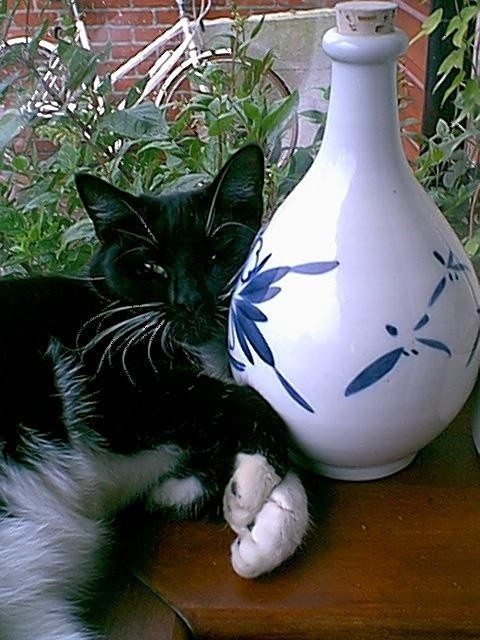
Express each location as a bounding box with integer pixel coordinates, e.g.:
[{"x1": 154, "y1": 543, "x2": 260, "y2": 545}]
[{"x1": 0, "y1": 141, "x2": 312, "y2": 640}]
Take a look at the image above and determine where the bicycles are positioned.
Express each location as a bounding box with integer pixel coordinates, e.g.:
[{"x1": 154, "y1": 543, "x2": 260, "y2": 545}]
[{"x1": 0, "y1": 0, "x2": 298, "y2": 188}]
